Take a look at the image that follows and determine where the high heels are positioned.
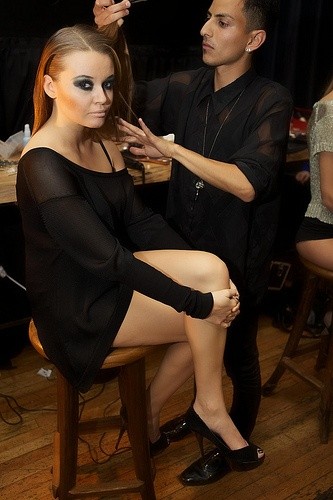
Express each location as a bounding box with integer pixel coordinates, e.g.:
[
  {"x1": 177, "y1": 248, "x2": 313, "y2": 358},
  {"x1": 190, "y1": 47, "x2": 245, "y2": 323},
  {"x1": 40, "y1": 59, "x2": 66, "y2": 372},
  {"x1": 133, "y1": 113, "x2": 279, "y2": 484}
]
[
  {"x1": 115, "y1": 406, "x2": 170, "y2": 452},
  {"x1": 185, "y1": 403, "x2": 265, "y2": 472}
]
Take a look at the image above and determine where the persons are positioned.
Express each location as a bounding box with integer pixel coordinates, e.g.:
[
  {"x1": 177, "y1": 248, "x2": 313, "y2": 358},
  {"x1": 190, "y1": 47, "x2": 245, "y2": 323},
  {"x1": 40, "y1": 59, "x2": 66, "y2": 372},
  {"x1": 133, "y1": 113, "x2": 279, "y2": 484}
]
[
  {"x1": 293, "y1": 79, "x2": 333, "y2": 333},
  {"x1": 15, "y1": 26, "x2": 266, "y2": 471},
  {"x1": 93, "y1": 0, "x2": 288, "y2": 486}
]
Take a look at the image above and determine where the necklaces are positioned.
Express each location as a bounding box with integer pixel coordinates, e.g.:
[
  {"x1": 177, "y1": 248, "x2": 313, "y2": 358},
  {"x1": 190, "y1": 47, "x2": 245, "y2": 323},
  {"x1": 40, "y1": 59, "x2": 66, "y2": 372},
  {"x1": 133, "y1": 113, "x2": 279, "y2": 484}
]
[{"x1": 193, "y1": 88, "x2": 245, "y2": 201}]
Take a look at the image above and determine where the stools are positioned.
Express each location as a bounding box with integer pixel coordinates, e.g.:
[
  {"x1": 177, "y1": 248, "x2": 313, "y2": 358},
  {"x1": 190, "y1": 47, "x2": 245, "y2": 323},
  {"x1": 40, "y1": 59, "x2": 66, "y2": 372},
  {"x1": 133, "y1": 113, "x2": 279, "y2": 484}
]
[
  {"x1": 262, "y1": 252, "x2": 333, "y2": 444},
  {"x1": 29, "y1": 318, "x2": 157, "y2": 500}
]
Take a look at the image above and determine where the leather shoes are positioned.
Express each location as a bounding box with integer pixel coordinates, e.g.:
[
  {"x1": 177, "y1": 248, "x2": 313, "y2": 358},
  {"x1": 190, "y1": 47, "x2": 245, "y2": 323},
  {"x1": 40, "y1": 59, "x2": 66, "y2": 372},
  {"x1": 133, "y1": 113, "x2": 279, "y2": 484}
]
[
  {"x1": 160, "y1": 414, "x2": 191, "y2": 441},
  {"x1": 177, "y1": 447, "x2": 232, "y2": 487}
]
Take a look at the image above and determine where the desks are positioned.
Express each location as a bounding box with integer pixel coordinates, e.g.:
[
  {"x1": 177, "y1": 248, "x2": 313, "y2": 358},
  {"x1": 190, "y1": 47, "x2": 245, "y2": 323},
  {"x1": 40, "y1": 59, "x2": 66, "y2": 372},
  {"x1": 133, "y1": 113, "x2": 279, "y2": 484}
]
[{"x1": 0, "y1": 147, "x2": 310, "y2": 204}]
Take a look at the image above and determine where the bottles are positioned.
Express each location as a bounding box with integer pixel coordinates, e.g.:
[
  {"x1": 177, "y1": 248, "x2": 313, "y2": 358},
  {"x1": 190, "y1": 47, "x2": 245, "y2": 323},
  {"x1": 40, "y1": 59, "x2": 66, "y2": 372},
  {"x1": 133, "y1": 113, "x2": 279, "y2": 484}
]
[{"x1": 22, "y1": 124, "x2": 31, "y2": 148}]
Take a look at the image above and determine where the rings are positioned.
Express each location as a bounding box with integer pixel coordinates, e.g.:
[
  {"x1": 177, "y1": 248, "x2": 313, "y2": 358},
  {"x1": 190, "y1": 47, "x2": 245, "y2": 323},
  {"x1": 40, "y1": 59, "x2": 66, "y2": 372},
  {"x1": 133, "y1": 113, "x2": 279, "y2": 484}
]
[{"x1": 233, "y1": 296, "x2": 240, "y2": 302}]
